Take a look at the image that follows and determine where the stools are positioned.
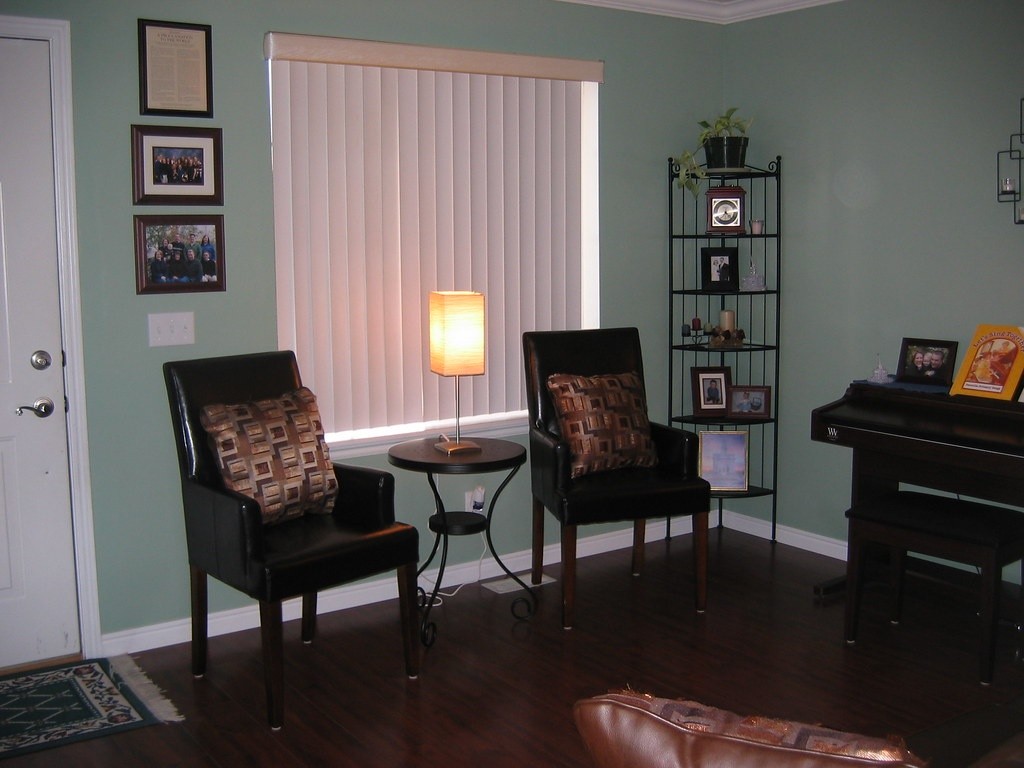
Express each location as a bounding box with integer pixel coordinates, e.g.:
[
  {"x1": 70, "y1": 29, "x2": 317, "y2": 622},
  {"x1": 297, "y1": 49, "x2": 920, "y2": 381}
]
[{"x1": 846, "y1": 491, "x2": 1024, "y2": 686}]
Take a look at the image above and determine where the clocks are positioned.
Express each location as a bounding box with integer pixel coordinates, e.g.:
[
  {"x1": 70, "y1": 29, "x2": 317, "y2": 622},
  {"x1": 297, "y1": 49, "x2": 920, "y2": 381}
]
[{"x1": 705, "y1": 185, "x2": 747, "y2": 235}]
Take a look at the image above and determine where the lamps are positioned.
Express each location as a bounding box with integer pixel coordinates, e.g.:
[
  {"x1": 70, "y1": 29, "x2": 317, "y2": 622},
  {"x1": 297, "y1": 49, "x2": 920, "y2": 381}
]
[{"x1": 429, "y1": 290, "x2": 482, "y2": 457}]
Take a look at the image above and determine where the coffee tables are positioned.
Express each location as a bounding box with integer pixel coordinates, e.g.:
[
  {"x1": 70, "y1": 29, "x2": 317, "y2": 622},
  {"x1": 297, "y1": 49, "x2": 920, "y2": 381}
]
[{"x1": 387, "y1": 437, "x2": 539, "y2": 645}]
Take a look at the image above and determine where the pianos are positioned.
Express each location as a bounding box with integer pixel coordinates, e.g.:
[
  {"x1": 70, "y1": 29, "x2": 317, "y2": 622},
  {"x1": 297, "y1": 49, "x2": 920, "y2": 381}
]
[{"x1": 809, "y1": 371, "x2": 1024, "y2": 599}]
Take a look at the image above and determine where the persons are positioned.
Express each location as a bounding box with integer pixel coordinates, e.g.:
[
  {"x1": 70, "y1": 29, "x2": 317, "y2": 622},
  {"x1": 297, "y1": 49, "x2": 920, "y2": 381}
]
[
  {"x1": 706, "y1": 380, "x2": 720, "y2": 404},
  {"x1": 711, "y1": 257, "x2": 729, "y2": 281},
  {"x1": 908, "y1": 350, "x2": 944, "y2": 377},
  {"x1": 151, "y1": 234, "x2": 217, "y2": 282},
  {"x1": 155, "y1": 154, "x2": 202, "y2": 183}
]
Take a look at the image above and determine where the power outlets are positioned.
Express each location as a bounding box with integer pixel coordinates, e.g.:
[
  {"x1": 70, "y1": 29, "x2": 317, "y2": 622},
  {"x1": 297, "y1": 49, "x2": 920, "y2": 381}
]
[{"x1": 465, "y1": 491, "x2": 480, "y2": 513}]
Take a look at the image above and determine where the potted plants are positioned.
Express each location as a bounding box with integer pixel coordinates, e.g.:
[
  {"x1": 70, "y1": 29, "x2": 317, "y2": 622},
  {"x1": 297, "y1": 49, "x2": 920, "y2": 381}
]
[{"x1": 672, "y1": 108, "x2": 752, "y2": 198}]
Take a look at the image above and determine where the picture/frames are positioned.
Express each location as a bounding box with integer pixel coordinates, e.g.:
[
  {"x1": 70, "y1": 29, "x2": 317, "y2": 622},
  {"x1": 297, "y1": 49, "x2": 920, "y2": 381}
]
[
  {"x1": 699, "y1": 246, "x2": 740, "y2": 292},
  {"x1": 690, "y1": 365, "x2": 733, "y2": 418},
  {"x1": 895, "y1": 336, "x2": 958, "y2": 388},
  {"x1": 725, "y1": 385, "x2": 772, "y2": 420},
  {"x1": 132, "y1": 214, "x2": 227, "y2": 295},
  {"x1": 698, "y1": 429, "x2": 749, "y2": 491},
  {"x1": 130, "y1": 124, "x2": 224, "y2": 206}
]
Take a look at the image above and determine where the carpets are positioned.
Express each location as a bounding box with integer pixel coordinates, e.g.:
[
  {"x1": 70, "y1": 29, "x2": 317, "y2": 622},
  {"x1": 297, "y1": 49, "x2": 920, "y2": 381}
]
[{"x1": 0, "y1": 652, "x2": 184, "y2": 759}]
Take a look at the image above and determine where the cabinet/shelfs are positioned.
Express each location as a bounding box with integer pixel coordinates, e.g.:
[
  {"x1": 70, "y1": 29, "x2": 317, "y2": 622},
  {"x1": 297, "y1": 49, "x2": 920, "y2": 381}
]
[{"x1": 668, "y1": 157, "x2": 780, "y2": 548}]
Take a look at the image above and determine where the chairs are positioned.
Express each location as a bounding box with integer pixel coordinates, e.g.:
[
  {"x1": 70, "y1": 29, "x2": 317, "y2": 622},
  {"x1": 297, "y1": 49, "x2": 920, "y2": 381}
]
[
  {"x1": 522, "y1": 325, "x2": 709, "y2": 628},
  {"x1": 163, "y1": 350, "x2": 421, "y2": 728}
]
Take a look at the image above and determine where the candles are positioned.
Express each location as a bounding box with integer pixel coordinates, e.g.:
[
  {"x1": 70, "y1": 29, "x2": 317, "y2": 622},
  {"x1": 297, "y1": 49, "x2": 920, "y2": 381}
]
[
  {"x1": 705, "y1": 323, "x2": 712, "y2": 332},
  {"x1": 682, "y1": 323, "x2": 690, "y2": 335},
  {"x1": 752, "y1": 220, "x2": 763, "y2": 234},
  {"x1": 1003, "y1": 179, "x2": 1014, "y2": 191},
  {"x1": 720, "y1": 310, "x2": 735, "y2": 330},
  {"x1": 692, "y1": 317, "x2": 701, "y2": 330}
]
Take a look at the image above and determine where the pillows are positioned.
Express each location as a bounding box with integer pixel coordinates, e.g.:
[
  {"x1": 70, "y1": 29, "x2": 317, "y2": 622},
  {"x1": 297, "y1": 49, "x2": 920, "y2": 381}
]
[
  {"x1": 575, "y1": 694, "x2": 925, "y2": 768},
  {"x1": 551, "y1": 369, "x2": 657, "y2": 480},
  {"x1": 199, "y1": 386, "x2": 339, "y2": 520}
]
